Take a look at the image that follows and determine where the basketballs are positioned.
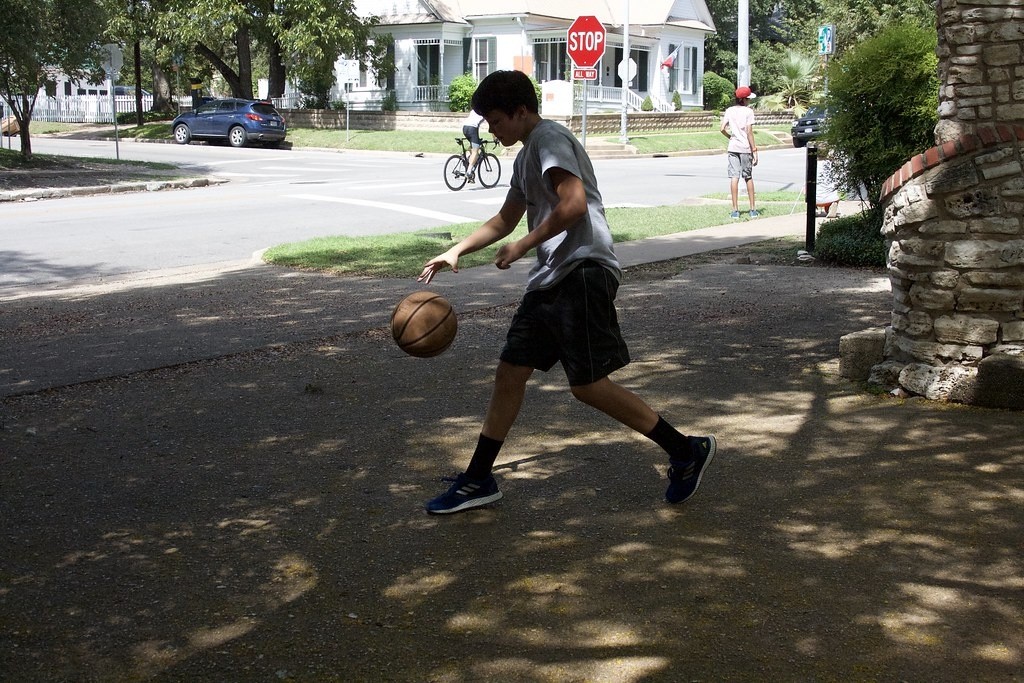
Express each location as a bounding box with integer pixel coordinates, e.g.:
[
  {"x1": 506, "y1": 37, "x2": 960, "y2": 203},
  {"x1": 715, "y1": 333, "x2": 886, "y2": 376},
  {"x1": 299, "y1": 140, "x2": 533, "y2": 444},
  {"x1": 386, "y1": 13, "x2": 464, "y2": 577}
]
[{"x1": 390, "y1": 291, "x2": 458, "y2": 358}]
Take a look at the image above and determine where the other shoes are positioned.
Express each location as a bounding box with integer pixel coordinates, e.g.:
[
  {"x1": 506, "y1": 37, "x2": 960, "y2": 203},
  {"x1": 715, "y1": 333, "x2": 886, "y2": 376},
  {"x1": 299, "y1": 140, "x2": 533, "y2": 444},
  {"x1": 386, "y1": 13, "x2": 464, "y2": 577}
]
[
  {"x1": 749, "y1": 210, "x2": 760, "y2": 217},
  {"x1": 465, "y1": 174, "x2": 476, "y2": 183},
  {"x1": 730, "y1": 211, "x2": 740, "y2": 218}
]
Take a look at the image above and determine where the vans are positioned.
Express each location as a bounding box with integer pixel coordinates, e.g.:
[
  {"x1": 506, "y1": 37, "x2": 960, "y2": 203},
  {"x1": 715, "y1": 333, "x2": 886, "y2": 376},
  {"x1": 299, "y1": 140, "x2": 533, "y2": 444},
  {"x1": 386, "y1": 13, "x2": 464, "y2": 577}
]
[{"x1": 791, "y1": 104, "x2": 831, "y2": 148}]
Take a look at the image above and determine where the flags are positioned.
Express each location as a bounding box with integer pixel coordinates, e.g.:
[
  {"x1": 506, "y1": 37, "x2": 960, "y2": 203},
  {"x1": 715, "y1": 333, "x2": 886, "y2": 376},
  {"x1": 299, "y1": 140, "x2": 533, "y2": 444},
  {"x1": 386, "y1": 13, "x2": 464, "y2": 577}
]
[{"x1": 660, "y1": 43, "x2": 682, "y2": 70}]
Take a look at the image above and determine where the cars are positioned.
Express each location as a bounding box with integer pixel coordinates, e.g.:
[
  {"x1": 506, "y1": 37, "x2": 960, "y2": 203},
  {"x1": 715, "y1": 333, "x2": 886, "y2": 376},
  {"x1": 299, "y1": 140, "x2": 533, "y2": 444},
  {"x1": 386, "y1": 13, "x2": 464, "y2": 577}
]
[{"x1": 112, "y1": 86, "x2": 150, "y2": 112}]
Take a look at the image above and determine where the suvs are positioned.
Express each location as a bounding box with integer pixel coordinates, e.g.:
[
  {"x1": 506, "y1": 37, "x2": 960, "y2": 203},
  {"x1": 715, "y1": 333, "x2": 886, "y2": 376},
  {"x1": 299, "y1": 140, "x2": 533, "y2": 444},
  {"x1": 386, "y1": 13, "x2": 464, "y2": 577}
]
[{"x1": 171, "y1": 97, "x2": 286, "y2": 146}]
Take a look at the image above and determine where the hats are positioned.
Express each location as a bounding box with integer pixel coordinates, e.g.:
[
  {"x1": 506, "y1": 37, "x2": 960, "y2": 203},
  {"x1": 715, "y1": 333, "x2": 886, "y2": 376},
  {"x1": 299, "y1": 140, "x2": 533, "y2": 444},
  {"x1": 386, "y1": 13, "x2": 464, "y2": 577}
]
[{"x1": 736, "y1": 86, "x2": 756, "y2": 99}]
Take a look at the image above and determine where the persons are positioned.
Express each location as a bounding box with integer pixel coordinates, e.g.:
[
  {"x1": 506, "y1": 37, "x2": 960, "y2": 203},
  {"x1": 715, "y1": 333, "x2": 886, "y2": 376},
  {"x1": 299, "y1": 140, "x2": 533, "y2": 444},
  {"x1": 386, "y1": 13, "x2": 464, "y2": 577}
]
[
  {"x1": 417, "y1": 70, "x2": 718, "y2": 515},
  {"x1": 720, "y1": 86, "x2": 759, "y2": 219},
  {"x1": 463, "y1": 109, "x2": 486, "y2": 179}
]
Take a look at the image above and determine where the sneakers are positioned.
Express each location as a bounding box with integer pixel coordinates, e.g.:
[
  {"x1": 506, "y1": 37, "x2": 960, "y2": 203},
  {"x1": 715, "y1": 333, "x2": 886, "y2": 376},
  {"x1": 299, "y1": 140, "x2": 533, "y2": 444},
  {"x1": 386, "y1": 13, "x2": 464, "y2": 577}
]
[
  {"x1": 665, "y1": 435, "x2": 717, "y2": 503},
  {"x1": 425, "y1": 471, "x2": 504, "y2": 514}
]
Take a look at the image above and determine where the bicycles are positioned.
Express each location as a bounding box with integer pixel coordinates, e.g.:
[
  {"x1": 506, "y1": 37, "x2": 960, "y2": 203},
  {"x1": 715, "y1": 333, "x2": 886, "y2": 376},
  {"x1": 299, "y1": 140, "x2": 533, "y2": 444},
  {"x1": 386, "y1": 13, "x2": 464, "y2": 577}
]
[{"x1": 443, "y1": 137, "x2": 501, "y2": 190}]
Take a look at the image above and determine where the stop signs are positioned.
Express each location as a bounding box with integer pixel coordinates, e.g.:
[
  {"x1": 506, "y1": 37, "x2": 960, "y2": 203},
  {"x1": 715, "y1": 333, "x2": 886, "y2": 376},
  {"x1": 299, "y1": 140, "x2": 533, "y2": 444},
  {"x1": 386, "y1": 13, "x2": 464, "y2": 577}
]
[{"x1": 565, "y1": 15, "x2": 606, "y2": 68}]
